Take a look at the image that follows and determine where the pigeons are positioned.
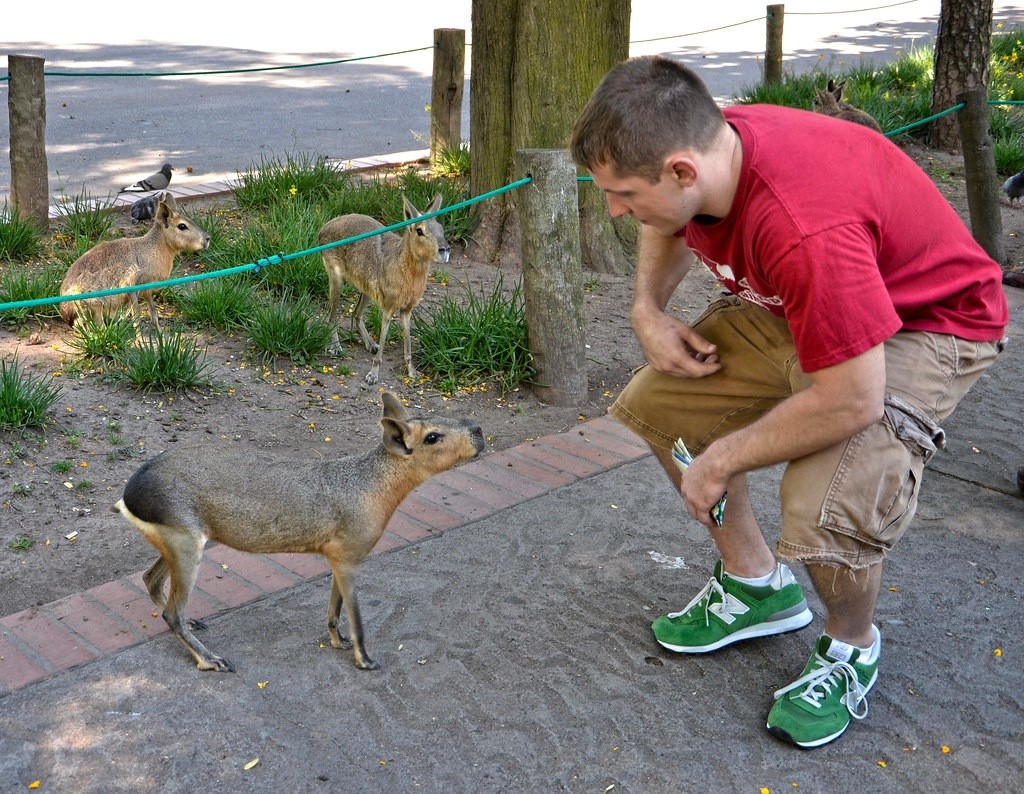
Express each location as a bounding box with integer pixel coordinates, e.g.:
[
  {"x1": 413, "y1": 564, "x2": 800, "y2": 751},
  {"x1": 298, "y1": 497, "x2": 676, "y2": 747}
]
[{"x1": 117, "y1": 164, "x2": 174, "y2": 220}]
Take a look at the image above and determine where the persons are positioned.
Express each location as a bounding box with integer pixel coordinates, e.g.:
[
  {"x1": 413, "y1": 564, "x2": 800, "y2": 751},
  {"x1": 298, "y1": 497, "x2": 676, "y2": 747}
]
[{"x1": 570, "y1": 55, "x2": 1010, "y2": 751}]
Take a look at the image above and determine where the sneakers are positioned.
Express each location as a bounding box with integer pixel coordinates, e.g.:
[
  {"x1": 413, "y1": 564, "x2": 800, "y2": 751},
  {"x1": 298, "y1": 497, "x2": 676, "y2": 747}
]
[
  {"x1": 651, "y1": 558, "x2": 813, "y2": 654},
  {"x1": 765, "y1": 621, "x2": 884, "y2": 748}
]
